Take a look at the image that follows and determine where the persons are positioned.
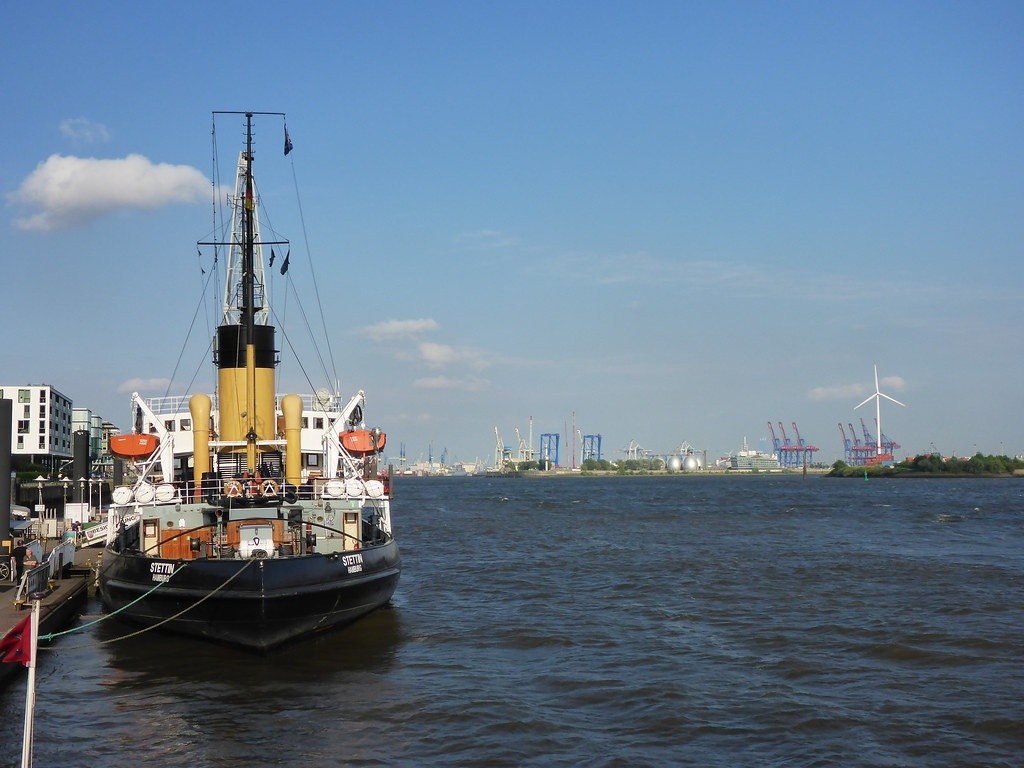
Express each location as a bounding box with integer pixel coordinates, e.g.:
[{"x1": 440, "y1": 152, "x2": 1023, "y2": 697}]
[
  {"x1": 11, "y1": 539, "x2": 37, "y2": 593},
  {"x1": 261, "y1": 462, "x2": 273, "y2": 478},
  {"x1": 72, "y1": 521, "x2": 83, "y2": 539}
]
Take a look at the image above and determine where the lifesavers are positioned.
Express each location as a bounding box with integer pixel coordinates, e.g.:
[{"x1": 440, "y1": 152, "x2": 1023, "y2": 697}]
[
  {"x1": 352, "y1": 539, "x2": 357, "y2": 544},
  {"x1": 224, "y1": 481, "x2": 242, "y2": 497},
  {"x1": 260, "y1": 480, "x2": 278, "y2": 497}
]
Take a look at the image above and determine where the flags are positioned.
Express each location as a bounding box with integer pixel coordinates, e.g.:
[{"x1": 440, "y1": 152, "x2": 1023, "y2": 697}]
[
  {"x1": 280, "y1": 250, "x2": 290, "y2": 275},
  {"x1": 284, "y1": 125, "x2": 293, "y2": 156},
  {"x1": 269, "y1": 248, "x2": 275, "y2": 267}
]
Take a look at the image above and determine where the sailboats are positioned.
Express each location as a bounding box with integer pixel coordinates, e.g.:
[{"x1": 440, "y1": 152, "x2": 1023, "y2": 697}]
[{"x1": 95, "y1": 109, "x2": 402, "y2": 652}]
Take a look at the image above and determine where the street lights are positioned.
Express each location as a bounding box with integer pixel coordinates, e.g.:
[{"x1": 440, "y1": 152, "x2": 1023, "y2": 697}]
[
  {"x1": 58, "y1": 476, "x2": 73, "y2": 521},
  {"x1": 33, "y1": 475, "x2": 49, "y2": 540},
  {"x1": 87, "y1": 477, "x2": 94, "y2": 522},
  {"x1": 77, "y1": 477, "x2": 87, "y2": 529},
  {"x1": 96, "y1": 477, "x2": 104, "y2": 516}
]
